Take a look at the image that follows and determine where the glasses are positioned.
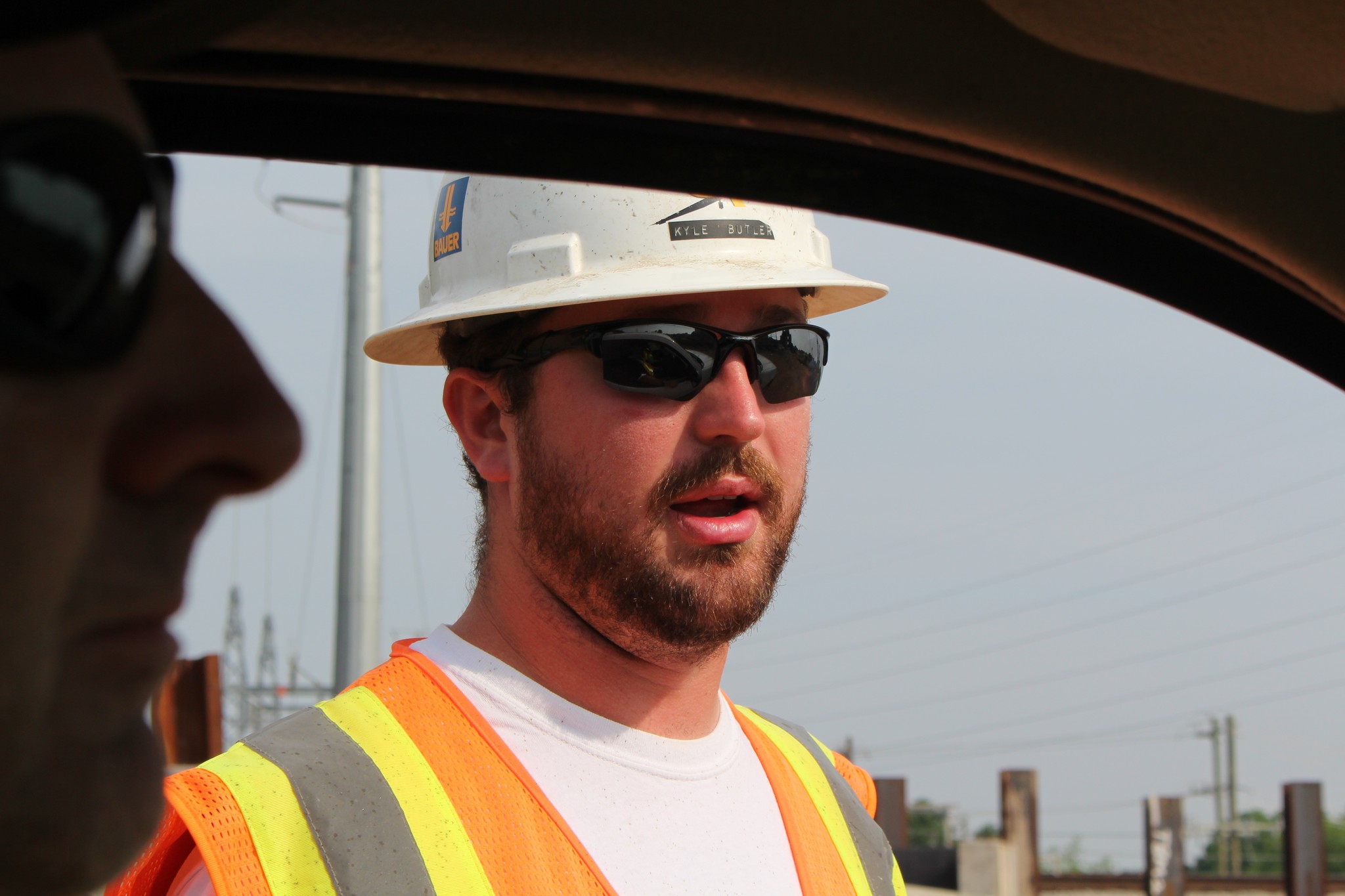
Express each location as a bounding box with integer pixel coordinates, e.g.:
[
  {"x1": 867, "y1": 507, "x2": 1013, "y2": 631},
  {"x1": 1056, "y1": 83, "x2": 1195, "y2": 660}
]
[
  {"x1": 481, "y1": 315, "x2": 831, "y2": 404},
  {"x1": 0, "y1": 138, "x2": 173, "y2": 382}
]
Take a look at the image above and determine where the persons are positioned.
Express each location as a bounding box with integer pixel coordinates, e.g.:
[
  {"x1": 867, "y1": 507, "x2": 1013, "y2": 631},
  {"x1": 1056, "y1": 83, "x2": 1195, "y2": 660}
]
[
  {"x1": 0, "y1": 0, "x2": 308, "y2": 896},
  {"x1": 129, "y1": 150, "x2": 929, "y2": 896}
]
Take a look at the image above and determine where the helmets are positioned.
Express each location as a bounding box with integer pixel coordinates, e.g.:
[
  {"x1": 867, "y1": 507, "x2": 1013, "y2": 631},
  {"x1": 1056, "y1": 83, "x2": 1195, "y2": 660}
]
[{"x1": 363, "y1": 169, "x2": 889, "y2": 366}]
